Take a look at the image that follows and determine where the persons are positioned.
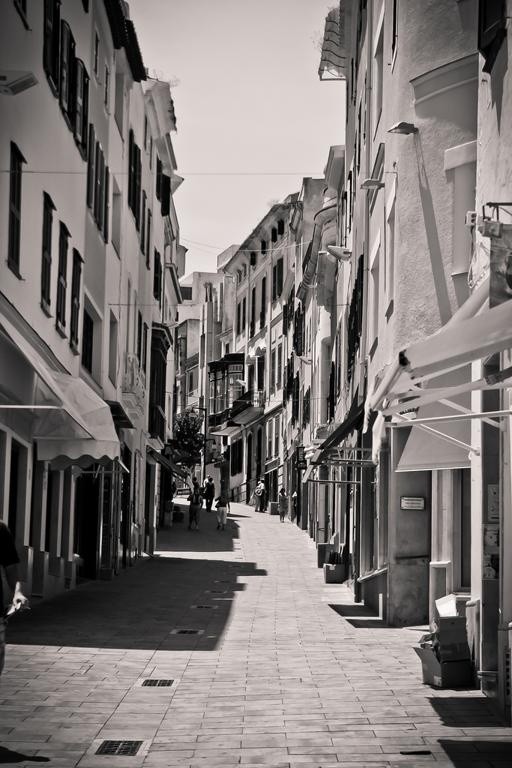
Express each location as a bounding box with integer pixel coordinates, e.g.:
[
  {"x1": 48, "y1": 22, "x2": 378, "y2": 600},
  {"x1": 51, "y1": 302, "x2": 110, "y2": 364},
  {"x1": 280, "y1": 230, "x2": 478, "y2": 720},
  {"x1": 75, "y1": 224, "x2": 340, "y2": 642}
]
[
  {"x1": 1, "y1": 520, "x2": 21, "y2": 674},
  {"x1": 192, "y1": 473, "x2": 199, "y2": 494},
  {"x1": 278, "y1": 487, "x2": 288, "y2": 523},
  {"x1": 203, "y1": 474, "x2": 211, "y2": 498},
  {"x1": 203, "y1": 478, "x2": 215, "y2": 513},
  {"x1": 253, "y1": 484, "x2": 266, "y2": 512},
  {"x1": 186, "y1": 486, "x2": 203, "y2": 532},
  {"x1": 214, "y1": 488, "x2": 231, "y2": 531}
]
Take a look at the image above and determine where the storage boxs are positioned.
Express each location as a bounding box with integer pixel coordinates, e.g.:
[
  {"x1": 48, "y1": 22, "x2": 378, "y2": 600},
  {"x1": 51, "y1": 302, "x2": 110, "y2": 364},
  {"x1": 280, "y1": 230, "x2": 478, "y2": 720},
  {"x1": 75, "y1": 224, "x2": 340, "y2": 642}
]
[{"x1": 410, "y1": 594, "x2": 475, "y2": 689}]
